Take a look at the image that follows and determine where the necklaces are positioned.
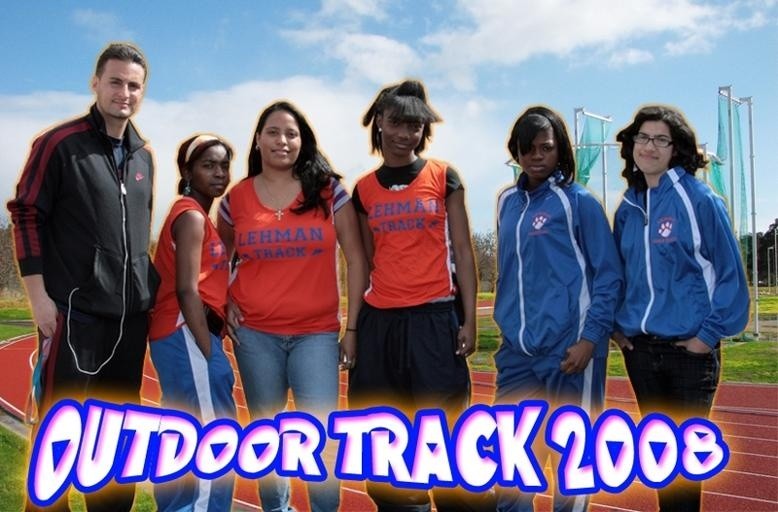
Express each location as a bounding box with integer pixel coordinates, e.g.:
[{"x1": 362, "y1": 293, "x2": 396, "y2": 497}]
[{"x1": 261, "y1": 174, "x2": 296, "y2": 221}]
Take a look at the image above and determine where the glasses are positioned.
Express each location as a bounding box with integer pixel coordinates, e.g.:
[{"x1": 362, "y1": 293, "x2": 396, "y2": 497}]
[{"x1": 633, "y1": 133, "x2": 673, "y2": 148}]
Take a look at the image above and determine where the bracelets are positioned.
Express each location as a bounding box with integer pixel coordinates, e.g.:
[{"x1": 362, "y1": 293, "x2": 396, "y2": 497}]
[{"x1": 347, "y1": 327, "x2": 358, "y2": 332}]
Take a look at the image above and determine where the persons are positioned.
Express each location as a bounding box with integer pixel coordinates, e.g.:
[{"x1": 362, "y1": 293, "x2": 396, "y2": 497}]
[
  {"x1": 7, "y1": 43, "x2": 162, "y2": 511},
  {"x1": 347, "y1": 79, "x2": 496, "y2": 512},
  {"x1": 493, "y1": 104, "x2": 626, "y2": 512},
  {"x1": 148, "y1": 134, "x2": 238, "y2": 512},
  {"x1": 217, "y1": 100, "x2": 369, "y2": 512},
  {"x1": 609, "y1": 103, "x2": 752, "y2": 512}
]
